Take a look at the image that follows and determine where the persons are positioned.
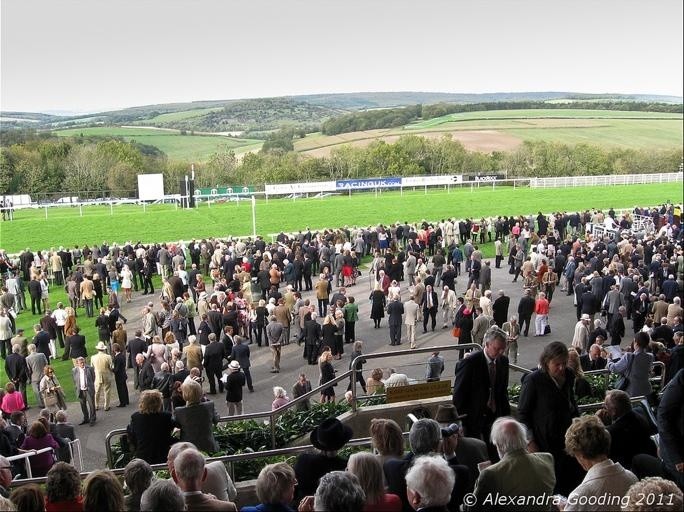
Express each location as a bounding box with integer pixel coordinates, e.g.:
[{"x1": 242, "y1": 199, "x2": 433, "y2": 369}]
[{"x1": 0, "y1": 199, "x2": 684, "y2": 512}]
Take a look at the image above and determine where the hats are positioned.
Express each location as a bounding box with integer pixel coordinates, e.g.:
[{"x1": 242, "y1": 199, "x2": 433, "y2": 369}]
[
  {"x1": 434, "y1": 404, "x2": 468, "y2": 423},
  {"x1": 95, "y1": 341, "x2": 107, "y2": 350},
  {"x1": 580, "y1": 314, "x2": 591, "y2": 321},
  {"x1": 462, "y1": 309, "x2": 471, "y2": 315},
  {"x1": 310, "y1": 418, "x2": 353, "y2": 451},
  {"x1": 228, "y1": 360, "x2": 241, "y2": 369}
]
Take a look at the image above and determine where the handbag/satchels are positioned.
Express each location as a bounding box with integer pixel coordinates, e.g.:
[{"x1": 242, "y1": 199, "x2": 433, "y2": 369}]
[
  {"x1": 614, "y1": 376, "x2": 630, "y2": 391},
  {"x1": 544, "y1": 324, "x2": 550, "y2": 334},
  {"x1": 44, "y1": 390, "x2": 57, "y2": 407},
  {"x1": 452, "y1": 327, "x2": 461, "y2": 337}
]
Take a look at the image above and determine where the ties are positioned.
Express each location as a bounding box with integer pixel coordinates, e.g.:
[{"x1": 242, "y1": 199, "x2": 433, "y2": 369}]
[{"x1": 489, "y1": 362, "x2": 497, "y2": 413}]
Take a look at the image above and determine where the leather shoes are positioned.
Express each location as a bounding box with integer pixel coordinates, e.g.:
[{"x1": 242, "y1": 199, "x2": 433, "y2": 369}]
[
  {"x1": 88, "y1": 421, "x2": 95, "y2": 428},
  {"x1": 78, "y1": 420, "x2": 90, "y2": 425}
]
